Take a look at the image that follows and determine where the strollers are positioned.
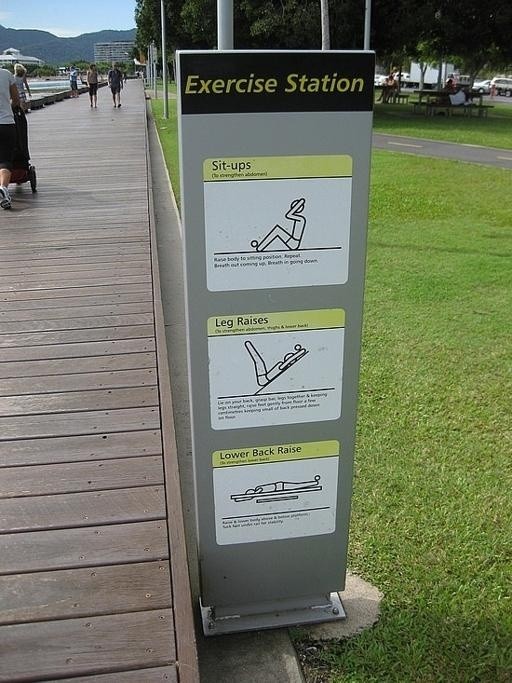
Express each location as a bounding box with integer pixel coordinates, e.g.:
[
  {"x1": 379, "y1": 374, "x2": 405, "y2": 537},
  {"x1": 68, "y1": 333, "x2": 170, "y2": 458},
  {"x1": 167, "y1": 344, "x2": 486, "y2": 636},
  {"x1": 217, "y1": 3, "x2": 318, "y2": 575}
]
[{"x1": 6, "y1": 102, "x2": 39, "y2": 194}]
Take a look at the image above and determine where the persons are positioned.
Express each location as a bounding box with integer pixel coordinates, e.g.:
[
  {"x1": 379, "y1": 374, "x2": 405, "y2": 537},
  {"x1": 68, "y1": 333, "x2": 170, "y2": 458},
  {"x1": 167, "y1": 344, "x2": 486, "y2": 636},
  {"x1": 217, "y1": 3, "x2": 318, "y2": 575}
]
[
  {"x1": 13, "y1": 62, "x2": 32, "y2": 109},
  {"x1": 66, "y1": 65, "x2": 79, "y2": 97},
  {"x1": 0, "y1": 67, "x2": 22, "y2": 208},
  {"x1": 86, "y1": 62, "x2": 99, "y2": 108},
  {"x1": 107, "y1": 60, "x2": 124, "y2": 108},
  {"x1": 377, "y1": 74, "x2": 498, "y2": 103}
]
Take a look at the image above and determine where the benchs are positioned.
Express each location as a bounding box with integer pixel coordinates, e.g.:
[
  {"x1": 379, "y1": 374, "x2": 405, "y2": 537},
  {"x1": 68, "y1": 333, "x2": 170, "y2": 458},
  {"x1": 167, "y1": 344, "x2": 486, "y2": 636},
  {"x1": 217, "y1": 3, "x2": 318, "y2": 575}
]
[
  {"x1": 409, "y1": 101, "x2": 494, "y2": 121},
  {"x1": 390, "y1": 95, "x2": 409, "y2": 105}
]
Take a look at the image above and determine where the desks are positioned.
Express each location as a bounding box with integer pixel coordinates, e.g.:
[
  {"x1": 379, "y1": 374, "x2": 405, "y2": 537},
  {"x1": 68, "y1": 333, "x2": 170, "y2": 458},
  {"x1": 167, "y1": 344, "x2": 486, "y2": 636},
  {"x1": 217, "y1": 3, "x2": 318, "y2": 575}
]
[
  {"x1": 375, "y1": 86, "x2": 396, "y2": 103},
  {"x1": 414, "y1": 90, "x2": 490, "y2": 117}
]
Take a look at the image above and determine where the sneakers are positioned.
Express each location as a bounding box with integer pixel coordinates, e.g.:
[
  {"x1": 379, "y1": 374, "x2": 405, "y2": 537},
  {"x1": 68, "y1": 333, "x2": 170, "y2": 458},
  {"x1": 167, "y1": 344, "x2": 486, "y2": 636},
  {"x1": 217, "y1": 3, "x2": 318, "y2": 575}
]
[{"x1": 0, "y1": 184, "x2": 13, "y2": 210}]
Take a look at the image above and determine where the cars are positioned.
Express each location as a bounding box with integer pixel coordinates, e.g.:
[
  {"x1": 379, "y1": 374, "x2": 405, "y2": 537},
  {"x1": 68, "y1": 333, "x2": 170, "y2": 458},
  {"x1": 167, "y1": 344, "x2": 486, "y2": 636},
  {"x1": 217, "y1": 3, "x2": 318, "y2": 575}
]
[{"x1": 373, "y1": 61, "x2": 512, "y2": 97}]
